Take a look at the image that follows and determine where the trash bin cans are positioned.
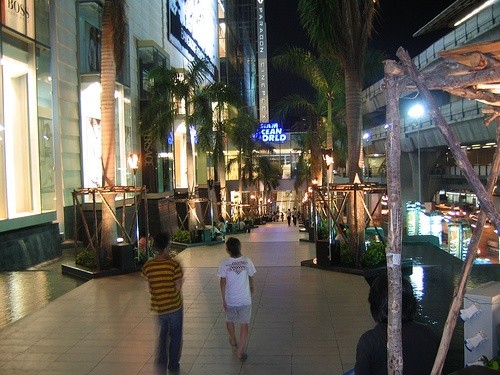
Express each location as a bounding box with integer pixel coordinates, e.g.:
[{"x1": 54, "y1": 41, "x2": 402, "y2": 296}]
[{"x1": 463, "y1": 281, "x2": 500, "y2": 368}]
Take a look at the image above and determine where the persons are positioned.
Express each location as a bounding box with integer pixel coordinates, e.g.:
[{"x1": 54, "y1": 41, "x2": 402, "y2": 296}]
[
  {"x1": 218, "y1": 237, "x2": 257, "y2": 361},
  {"x1": 286, "y1": 209, "x2": 291, "y2": 225},
  {"x1": 141, "y1": 232, "x2": 184, "y2": 375},
  {"x1": 214, "y1": 217, "x2": 226, "y2": 242},
  {"x1": 265, "y1": 211, "x2": 279, "y2": 222},
  {"x1": 335, "y1": 224, "x2": 344, "y2": 241},
  {"x1": 355, "y1": 275, "x2": 449, "y2": 375},
  {"x1": 139, "y1": 235, "x2": 153, "y2": 266},
  {"x1": 292, "y1": 208, "x2": 297, "y2": 226},
  {"x1": 281, "y1": 213, "x2": 284, "y2": 222},
  {"x1": 226, "y1": 211, "x2": 253, "y2": 233}
]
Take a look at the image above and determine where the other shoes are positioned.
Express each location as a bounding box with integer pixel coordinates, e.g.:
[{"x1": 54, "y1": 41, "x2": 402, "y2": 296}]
[
  {"x1": 229, "y1": 336, "x2": 237, "y2": 346},
  {"x1": 239, "y1": 352, "x2": 248, "y2": 360}
]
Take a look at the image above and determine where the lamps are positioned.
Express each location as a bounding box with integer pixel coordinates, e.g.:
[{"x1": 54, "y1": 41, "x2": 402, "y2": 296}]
[
  {"x1": 458, "y1": 304, "x2": 482, "y2": 323},
  {"x1": 466, "y1": 355, "x2": 490, "y2": 368},
  {"x1": 463, "y1": 332, "x2": 487, "y2": 352}
]
